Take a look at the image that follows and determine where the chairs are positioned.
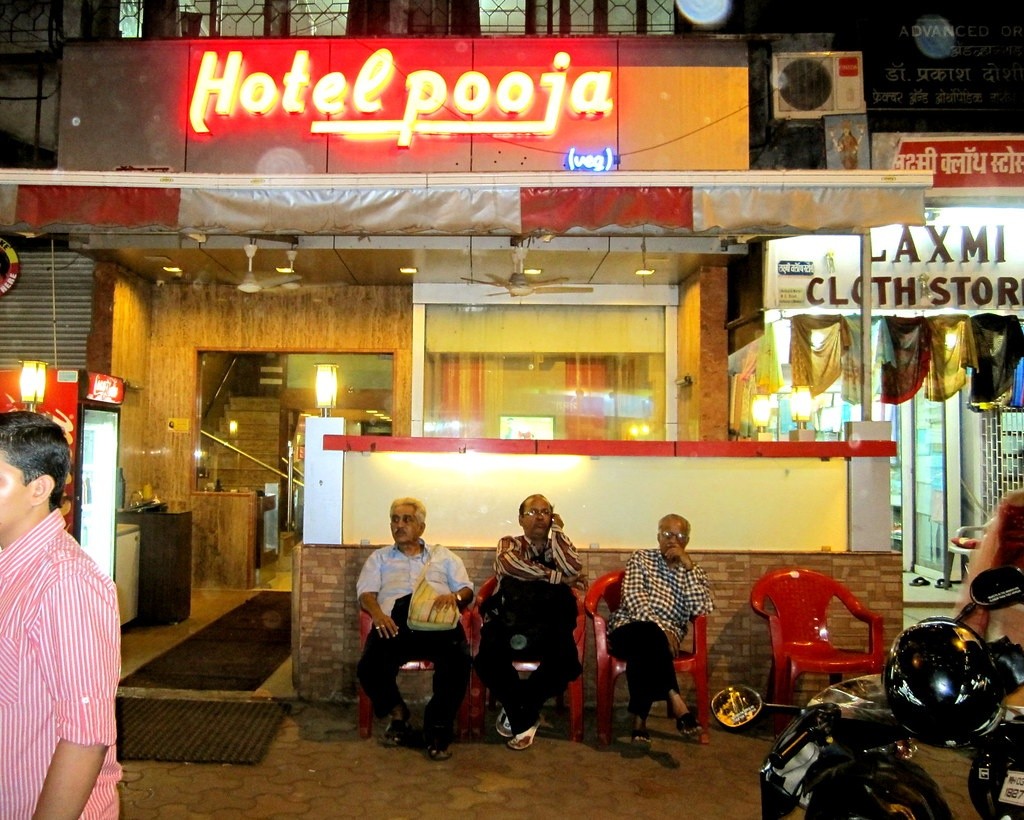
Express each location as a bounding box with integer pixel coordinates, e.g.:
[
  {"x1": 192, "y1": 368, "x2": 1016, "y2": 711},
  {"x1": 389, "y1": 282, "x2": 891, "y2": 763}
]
[
  {"x1": 948, "y1": 515, "x2": 999, "y2": 574},
  {"x1": 360, "y1": 567, "x2": 711, "y2": 750},
  {"x1": 750, "y1": 566, "x2": 887, "y2": 734}
]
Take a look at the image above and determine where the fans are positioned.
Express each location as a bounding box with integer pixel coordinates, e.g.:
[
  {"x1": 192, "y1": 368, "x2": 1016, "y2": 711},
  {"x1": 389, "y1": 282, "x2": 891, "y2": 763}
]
[
  {"x1": 459, "y1": 239, "x2": 594, "y2": 298},
  {"x1": 193, "y1": 245, "x2": 350, "y2": 293}
]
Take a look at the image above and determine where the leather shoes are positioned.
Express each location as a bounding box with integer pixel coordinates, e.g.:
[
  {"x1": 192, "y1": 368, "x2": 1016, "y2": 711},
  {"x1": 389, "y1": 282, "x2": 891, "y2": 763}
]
[
  {"x1": 427, "y1": 731, "x2": 451, "y2": 759},
  {"x1": 384, "y1": 702, "x2": 411, "y2": 745}
]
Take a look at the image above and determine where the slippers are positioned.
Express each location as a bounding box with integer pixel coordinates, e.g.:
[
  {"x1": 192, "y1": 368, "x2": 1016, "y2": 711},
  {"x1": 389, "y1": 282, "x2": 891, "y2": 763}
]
[
  {"x1": 935, "y1": 579, "x2": 952, "y2": 587},
  {"x1": 909, "y1": 577, "x2": 930, "y2": 585},
  {"x1": 631, "y1": 730, "x2": 652, "y2": 743},
  {"x1": 676, "y1": 712, "x2": 702, "y2": 736}
]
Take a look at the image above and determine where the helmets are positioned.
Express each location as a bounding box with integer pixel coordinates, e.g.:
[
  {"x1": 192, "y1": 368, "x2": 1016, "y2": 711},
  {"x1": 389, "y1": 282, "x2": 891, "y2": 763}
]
[{"x1": 883, "y1": 617, "x2": 1005, "y2": 750}]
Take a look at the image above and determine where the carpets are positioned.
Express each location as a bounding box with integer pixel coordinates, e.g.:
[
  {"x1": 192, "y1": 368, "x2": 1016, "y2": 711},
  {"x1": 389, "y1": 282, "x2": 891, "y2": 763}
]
[
  {"x1": 115, "y1": 696, "x2": 292, "y2": 766},
  {"x1": 120, "y1": 590, "x2": 292, "y2": 692}
]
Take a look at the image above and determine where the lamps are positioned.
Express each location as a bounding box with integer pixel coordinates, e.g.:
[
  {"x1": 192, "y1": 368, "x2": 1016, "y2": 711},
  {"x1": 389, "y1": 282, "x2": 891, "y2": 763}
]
[
  {"x1": 790, "y1": 384, "x2": 813, "y2": 429},
  {"x1": 314, "y1": 362, "x2": 339, "y2": 418},
  {"x1": 675, "y1": 375, "x2": 692, "y2": 385},
  {"x1": 751, "y1": 394, "x2": 771, "y2": 433},
  {"x1": 19, "y1": 359, "x2": 49, "y2": 413}
]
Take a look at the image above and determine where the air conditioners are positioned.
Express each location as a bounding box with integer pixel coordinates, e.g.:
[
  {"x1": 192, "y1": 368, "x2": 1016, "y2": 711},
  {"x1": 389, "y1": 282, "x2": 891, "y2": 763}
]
[{"x1": 772, "y1": 51, "x2": 866, "y2": 130}]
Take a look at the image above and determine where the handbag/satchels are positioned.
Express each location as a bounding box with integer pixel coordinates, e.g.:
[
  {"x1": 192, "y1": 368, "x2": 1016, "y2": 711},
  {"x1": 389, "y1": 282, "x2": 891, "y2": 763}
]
[
  {"x1": 478, "y1": 555, "x2": 579, "y2": 649},
  {"x1": 406, "y1": 543, "x2": 460, "y2": 631}
]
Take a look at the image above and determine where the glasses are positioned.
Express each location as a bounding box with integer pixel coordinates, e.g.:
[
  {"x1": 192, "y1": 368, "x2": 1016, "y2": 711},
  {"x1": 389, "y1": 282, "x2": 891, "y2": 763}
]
[
  {"x1": 660, "y1": 531, "x2": 689, "y2": 542},
  {"x1": 522, "y1": 509, "x2": 552, "y2": 517}
]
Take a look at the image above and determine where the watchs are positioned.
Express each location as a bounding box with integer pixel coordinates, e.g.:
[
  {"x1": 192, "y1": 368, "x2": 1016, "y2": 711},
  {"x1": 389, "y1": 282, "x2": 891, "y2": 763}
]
[{"x1": 454, "y1": 593, "x2": 462, "y2": 602}]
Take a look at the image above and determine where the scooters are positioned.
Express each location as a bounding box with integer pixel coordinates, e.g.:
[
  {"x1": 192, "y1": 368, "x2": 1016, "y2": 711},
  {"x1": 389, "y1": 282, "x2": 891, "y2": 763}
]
[{"x1": 708, "y1": 563, "x2": 1023, "y2": 820}]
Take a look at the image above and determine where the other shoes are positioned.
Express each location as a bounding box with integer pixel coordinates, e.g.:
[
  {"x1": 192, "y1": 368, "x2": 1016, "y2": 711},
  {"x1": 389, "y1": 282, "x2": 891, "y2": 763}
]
[
  {"x1": 496, "y1": 708, "x2": 513, "y2": 737},
  {"x1": 507, "y1": 721, "x2": 540, "y2": 749}
]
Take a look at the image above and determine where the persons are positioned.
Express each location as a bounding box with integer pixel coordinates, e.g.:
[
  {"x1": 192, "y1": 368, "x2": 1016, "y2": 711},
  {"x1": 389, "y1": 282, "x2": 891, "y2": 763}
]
[
  {"x1": 0, "y1": 411, "x2": 124, "y2": 820},
  {"x1": 604, "y1": 514, "x2": 715, "y2": 753},
  {"x1": 355, "y1": 496, "x2": 476, "y2": 761},
  {"x1": 476, "y1": 493, "x2": 591, "y2": 749},
  {"x1": 959, "y1": 487, "x2": 1024, "y2": 717}
]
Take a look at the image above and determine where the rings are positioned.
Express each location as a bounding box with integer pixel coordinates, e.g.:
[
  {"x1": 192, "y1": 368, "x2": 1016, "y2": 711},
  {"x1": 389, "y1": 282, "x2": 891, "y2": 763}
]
[
  {"x1": 376, "y1": 626, "x2": 379, "y2": 630},
  {"x1": 381, "y1": 625, "x2": 385, "y2": 628}
]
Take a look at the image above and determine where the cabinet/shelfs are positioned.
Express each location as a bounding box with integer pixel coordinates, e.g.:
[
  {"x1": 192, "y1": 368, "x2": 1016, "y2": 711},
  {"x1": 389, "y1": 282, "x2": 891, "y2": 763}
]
[{"x1": 114, "y1": 506, "x2": 193, "y2": 627}]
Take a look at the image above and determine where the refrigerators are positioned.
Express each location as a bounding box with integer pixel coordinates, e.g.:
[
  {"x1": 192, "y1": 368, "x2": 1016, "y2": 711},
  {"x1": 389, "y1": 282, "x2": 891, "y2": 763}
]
[{"x1": 0, "y1": 359, "x2": 124, "y2": 581}]
